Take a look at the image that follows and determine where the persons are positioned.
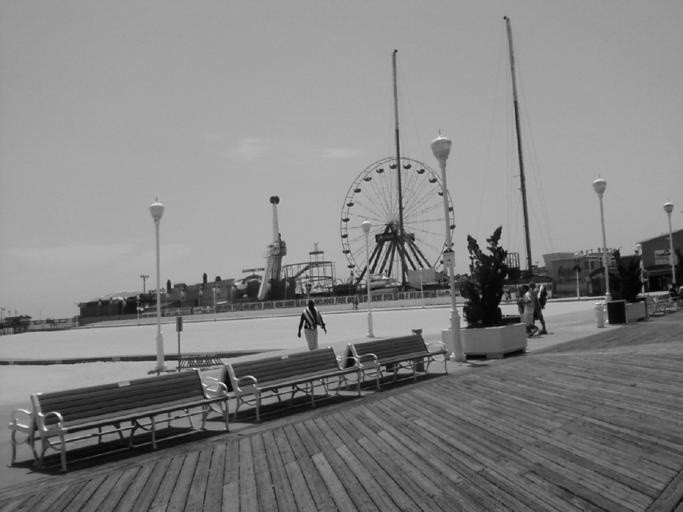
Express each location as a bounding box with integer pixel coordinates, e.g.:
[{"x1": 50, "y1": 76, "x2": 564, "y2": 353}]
[
  {"x1": 504, "y1": 279, "x2": 547, "y2": 338},
  {"x1": 355, "y1": 296, "x2": 359, "y2": 309},
  {"x1": 351, "y1": 297, "x2": 355, "y2": 307},
  {"x1": 669, "y1": 282, "x2": 679, "y2": 302},
  {"x1": 297, "y1": 298, "x2": 327, "y2": 350}
]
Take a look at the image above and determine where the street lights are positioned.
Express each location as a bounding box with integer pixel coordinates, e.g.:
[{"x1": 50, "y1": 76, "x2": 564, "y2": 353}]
[
  {"x1": 417, "y1": 267, "x2": 425, "y2": 309},
  {"x1": 360, "y1": 218, "x2": 376, "y2": 338},
  {"x1": 591, "y1": 176, "x2": 612, "y2": 301},
  {"x1": 574, "y1": 251, "x2": 580, "y2": 301},
  {"x1": 135, "y1": 294, "x2": 142, "y2": 327},
  {"x1": 662, "y1": 201, "x2": 677, "y2": 283},
  {"x1": 179, "y1": 286, "x2": 235, "y2": 322},
  {"x1": 148, "y1": 196, "x2": 166, "y2": 374},
  {"x1": 633, "y1": 243, "x2": 646, "y2": 295},
  {"x1": 429, "y1": 128, "x2": 467, "y2": 363}
]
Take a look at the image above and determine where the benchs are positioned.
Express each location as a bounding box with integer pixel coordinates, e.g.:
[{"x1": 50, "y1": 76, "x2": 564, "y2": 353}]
[
  {"x1": 4, "y1": 367, "x2": 222, "y2": 474},
  {"x1": 333, "y1": 333, "x2": 449, "y2": 395},
  {"x1": 205, "y1": 346, "x2": 366, "y2": 424}
]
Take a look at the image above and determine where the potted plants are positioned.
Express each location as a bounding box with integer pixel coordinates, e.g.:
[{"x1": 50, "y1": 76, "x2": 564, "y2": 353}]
[
  {"x1": 606, "y1": 248, "x2": 646, "y2": 324},
  {"x1": 441, "y1": 225, "x2": 528, "y2": 360}
]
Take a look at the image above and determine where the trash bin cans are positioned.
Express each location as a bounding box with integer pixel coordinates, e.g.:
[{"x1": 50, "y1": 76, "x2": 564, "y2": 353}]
[{"x1": 607, "y1": 300, "x2": 626, "y2": 324}]
